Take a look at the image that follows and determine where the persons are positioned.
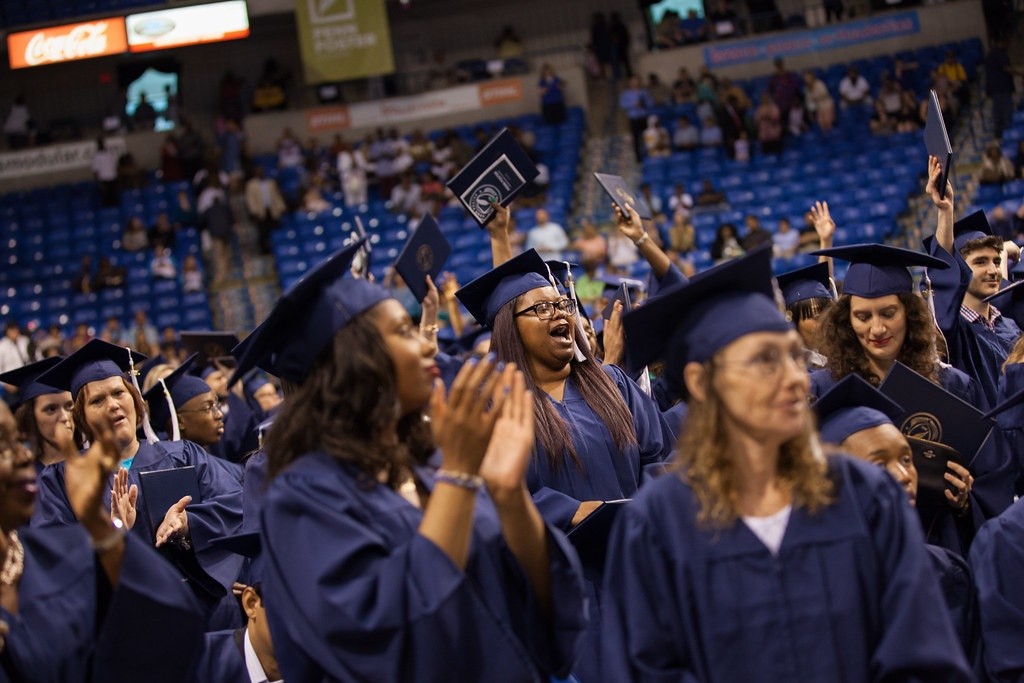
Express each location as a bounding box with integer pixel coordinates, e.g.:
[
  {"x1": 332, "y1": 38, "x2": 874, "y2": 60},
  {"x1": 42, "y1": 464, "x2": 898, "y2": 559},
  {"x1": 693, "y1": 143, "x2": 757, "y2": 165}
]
[
  {"x1": 0, "y1": 311, "x2": 285, "y2": 683},
  {"x1": 231, "y1": 156, "x2": 1024, "y2": 683},
  {"x1": 569, "y1": 0, "x2": 1024, "y2": 318},
  {"x1": 0, "y1": 0, "x2": 570, "y2": 293}
]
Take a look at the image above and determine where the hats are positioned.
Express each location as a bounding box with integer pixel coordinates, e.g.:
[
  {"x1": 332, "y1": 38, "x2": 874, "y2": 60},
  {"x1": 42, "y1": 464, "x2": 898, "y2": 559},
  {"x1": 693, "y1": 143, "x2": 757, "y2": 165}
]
[{"x1": 0, "y1": 208, "x2": 1023, "y2": 586}]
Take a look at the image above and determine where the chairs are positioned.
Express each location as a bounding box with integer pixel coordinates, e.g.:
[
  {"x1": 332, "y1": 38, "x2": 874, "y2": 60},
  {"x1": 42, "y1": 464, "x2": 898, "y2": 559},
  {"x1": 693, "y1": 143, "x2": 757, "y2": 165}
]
[{"x1": 0, "y1": 33, "x2": 988, "y2": 332}]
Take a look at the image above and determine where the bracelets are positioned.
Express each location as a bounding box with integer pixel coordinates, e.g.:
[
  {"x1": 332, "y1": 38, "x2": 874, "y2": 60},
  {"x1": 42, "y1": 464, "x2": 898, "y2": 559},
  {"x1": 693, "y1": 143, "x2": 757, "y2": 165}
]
[
  {"x1": 634, "y1": 232, "x2": 649, "y2": 246},
  {"x1": 435, "y1": 470, "x2": 484, "y2": 491}
]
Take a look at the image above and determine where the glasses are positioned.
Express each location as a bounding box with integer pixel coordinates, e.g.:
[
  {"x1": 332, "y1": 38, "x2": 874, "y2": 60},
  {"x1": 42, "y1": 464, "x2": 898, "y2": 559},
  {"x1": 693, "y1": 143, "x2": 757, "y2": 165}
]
[
  {"x1": 176, "y1": 402, "x2": 222, "y2": 413},
  {"x1": 513, "y1": 298, "x2": 576, "y2": 320}
]
